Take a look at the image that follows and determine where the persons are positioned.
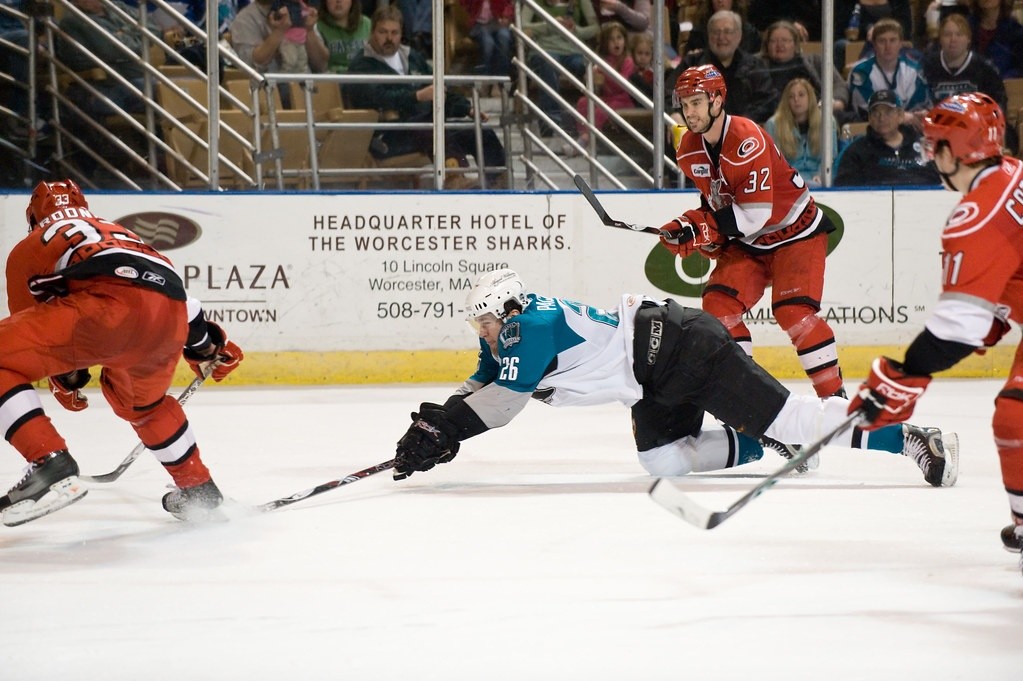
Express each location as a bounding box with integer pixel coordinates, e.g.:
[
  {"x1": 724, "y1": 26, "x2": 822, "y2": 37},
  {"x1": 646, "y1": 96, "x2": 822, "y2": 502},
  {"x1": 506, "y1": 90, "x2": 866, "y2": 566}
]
[
  {"x1": 450, "y1": 0, "x2": 1023, "y2": 157},
  {"x1": 832, "y1": 90, "x2": 942, "y2": 185},
  {"x1": 125, "y1": 0, "x2": 505, "y2": 173},
  {"x1": 0, "y1": 0, "x2": 158, "y2": 184},
  {"x1": 847, "y1": 92, "x2": 1023, "y2": 553},
  {"x1": 765, "y1": 78, "x2": 838, "y2": 183},
  {"x1": 0, "y1": 179, "x2": 245, "y2": 526},
  {"x1": 659, "y1": 62, "x2": 845, "y2": 400},
  {"x1": 394, "y1": 268, "x2": 961, "y2": 488}
]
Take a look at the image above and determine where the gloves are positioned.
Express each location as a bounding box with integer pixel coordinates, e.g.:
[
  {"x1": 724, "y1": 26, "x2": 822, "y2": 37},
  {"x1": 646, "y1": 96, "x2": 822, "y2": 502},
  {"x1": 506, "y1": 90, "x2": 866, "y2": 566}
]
[
  {"x1": 846, "y1": 357, "x2": 933, "y2": 431},
  {"x1": 48, "y1": 368, "x2": 91, "y2": 411},
  {"x1": 394, "y1": 416, "x2": 461, "y2": 478},
  {"x1": 659, "y1": 217, "x2": 698, "y2": 257},
  {"x1": 182, "y1": 323, "x2": 243, "y2": 381},
  {"x1": 701, "y1": 242, "x2": 724, "y2": 260}
]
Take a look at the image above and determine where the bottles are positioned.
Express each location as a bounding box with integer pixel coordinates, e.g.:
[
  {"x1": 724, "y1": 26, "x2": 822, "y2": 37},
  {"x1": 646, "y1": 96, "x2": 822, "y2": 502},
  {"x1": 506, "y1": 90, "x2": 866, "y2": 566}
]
[
  {"x1": 846, "y1": 3, "x2": 861, "y2": 40},
  {"x1": 566, "y1": 0, "x2": 575, "y2": 17}
]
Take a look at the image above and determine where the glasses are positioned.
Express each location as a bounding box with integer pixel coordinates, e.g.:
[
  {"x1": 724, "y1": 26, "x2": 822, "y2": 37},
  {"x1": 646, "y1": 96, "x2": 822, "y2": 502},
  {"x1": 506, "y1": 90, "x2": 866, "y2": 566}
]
[{"x1": 712, "y1": 29, "x2": 736, "y2": 36}]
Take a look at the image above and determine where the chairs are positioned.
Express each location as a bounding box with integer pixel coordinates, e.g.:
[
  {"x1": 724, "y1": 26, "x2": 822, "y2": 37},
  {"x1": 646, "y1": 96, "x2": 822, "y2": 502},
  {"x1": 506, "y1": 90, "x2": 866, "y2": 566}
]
[
  {"x1": 49, "y1": 41, "x2": 432, "y2": 190},
  {"x1": 755, "y1": 42, "x2": 1023, "y2": 141},
  {"x1": 584, "y1": 65, "x2": 653, "y2": 144}
]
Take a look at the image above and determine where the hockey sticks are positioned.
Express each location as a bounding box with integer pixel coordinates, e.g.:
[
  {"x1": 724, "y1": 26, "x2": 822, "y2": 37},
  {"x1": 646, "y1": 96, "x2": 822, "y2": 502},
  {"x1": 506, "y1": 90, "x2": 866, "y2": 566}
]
[
  {"x1": 77, "y1": 355, "x2": 229, "y2": 483},
  {"x1": 160, "y1": 453, "x2": 405, "y2": 524},
  {"x1": 573, "y1": 174, "x2": 685, "y2": 240}
]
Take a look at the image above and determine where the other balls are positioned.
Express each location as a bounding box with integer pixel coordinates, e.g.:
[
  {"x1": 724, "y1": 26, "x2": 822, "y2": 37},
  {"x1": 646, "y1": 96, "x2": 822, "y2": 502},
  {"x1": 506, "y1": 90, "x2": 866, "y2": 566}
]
[{"x1": 161, "y1": 491, "x2": 189, "y2": 513}]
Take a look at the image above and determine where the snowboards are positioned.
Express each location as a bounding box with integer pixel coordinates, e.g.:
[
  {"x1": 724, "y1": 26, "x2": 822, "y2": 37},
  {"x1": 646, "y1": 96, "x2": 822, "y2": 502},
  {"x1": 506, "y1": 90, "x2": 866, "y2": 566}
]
[{"x1": 645, "y1": 407, "x2": 867, "y2": 530}]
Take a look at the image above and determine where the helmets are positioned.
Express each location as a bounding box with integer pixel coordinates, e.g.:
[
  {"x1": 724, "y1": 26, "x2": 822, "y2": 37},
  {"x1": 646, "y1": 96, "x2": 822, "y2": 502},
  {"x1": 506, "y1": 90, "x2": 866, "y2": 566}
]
[
  {"x1": 923, "y1": 92, "x2": 1006, "y2": 166},
  {"x1": 675, "y1": 64, "x2": 727, "y2": 102},
  {"x1": 465, "y1": 268, "x2": 526, "y2": 356},
  {"x1": 26, "y1": 179, "x2": 89, "y2": 233}
]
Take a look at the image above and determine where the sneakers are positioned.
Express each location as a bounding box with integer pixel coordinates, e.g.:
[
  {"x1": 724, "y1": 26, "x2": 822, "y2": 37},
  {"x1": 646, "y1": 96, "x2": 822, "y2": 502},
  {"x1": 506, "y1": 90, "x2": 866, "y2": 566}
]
[
  {"x1": 1001, "y1": 524, "x2": 1023, "y2": 553},
  {"x1": 758, "y1": 436, "x2": 808, "y2": 473},
  {"x1": 0, "y1": 450, "x2": 84, "y2": 526},
  {"x1": 902, "y1": 424, "x2": 959, "y2": 487},
  {"x1": 162, "y1": 481, "x2": 230, "y2": 524}
]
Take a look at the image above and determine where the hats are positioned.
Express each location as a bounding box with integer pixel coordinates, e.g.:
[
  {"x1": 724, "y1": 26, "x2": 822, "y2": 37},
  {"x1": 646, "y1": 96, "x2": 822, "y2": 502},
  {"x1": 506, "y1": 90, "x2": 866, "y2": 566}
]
[{"x1": 869, "y1": 89, "x2": 901, "y2": 111}]
